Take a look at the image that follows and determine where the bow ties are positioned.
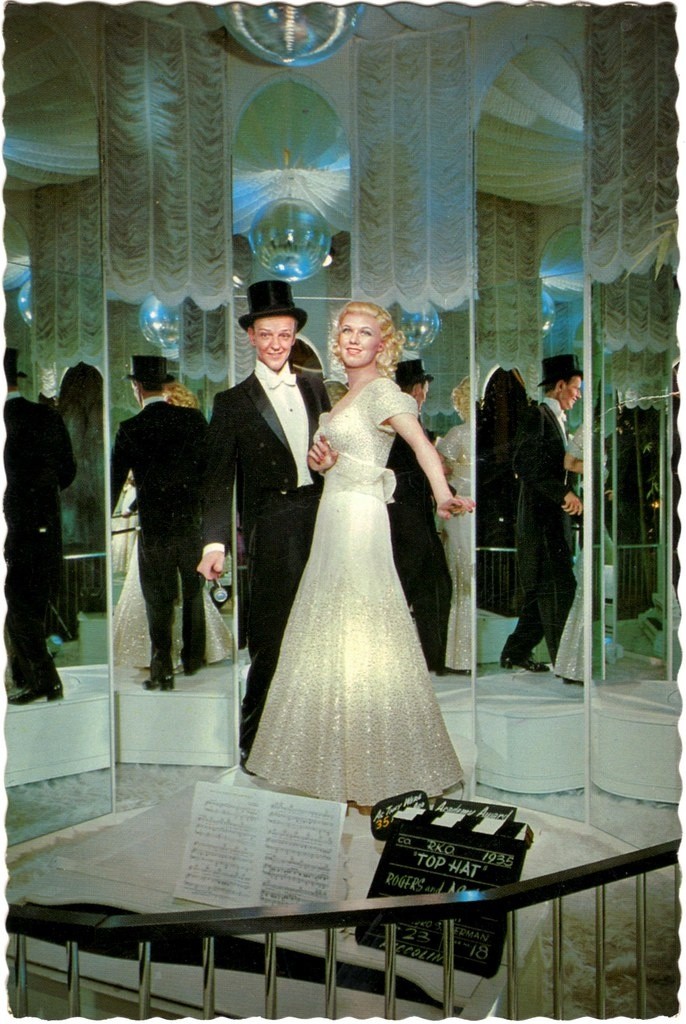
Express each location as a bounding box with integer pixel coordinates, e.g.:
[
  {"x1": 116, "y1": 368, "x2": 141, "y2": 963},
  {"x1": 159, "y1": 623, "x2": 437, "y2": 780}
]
[
  {"x1": 264, "y1": 368, "x2": 296, "y2": 389},
  {"x1": 557, "y1": 409, "x2": 567, "y2": 421}
]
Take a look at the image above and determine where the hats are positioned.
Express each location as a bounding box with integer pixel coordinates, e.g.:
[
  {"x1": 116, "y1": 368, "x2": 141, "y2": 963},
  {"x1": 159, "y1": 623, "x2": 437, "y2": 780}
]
[
  {"x1": 537, "y1": 355, "x2": 583, "y2": 386},
  {"x1": 396, "y1": 358, "x2": 435, "y2": 386},
  {"x1": 4, "y1": 347, "x2": 28, "y2": 377},
  {"x1": 122, "y1": 355, "x2": 175, "y2": 384},
  {"x1": 238, "y1": 280, "x2": 307, "y2": 334}
]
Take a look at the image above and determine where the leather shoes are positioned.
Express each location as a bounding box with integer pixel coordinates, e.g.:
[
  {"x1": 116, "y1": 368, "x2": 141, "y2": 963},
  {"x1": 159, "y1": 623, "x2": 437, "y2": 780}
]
[
  {"x1": 8, "y1": 681, "x2": 64, "y2": 707},
  {"x1": 184, "y1": 658, "x2": 208, "y2": 675},
  {"x1": 140, "y1": 673, "x2": 173, "y2": 691},
  {"x1": 498, "y1": 650, "x2": 550, "y2": 672}
]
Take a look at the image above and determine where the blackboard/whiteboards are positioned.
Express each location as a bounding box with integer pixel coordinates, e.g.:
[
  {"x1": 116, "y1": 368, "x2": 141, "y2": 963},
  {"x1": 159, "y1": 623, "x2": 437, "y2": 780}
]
[{"x1": 352, "y1": 819, "x2": 529, "y2": 981}]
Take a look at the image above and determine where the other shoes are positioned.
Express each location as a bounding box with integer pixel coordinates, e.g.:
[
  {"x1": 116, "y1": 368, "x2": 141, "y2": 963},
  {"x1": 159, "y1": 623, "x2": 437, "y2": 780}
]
[
  {"x1": 437, "y1": 667, "x2": 472, "y2": 676},
  {"x1": 239, "y1": 748, "x2": 255, "y2": 776}
]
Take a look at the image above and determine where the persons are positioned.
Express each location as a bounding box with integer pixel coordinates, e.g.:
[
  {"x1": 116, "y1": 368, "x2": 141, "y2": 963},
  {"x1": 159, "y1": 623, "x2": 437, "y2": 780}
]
[
  {"x1": 196, "y1": 280, "x2": 331, "y2": 775},
  {"x1": 2, "y1": 344, "x2": 235, "y2": 707},
  {"x1": 387, "y1": 354, "x2": 586, "y2": 687},
  {"x1": 247, "y1": 303, "x2": 474, "y2": 809}
]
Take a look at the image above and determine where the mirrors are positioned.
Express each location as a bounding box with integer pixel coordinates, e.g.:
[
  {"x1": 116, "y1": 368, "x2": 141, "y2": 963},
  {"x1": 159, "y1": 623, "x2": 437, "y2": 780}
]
[
  {"x1": 353, "y1": 2, "x2": 476, "y2": 786},
  {"x1": 474, "y1": 0, "x2": 588, "y2": 796},
  {"x1": 225, "y1": 0, "x2": 358, "y2": 764},
  {"x1": 3, "y1": 0, "x2": 113, "y2": 848},
  {"x1": 104, "y1": 3, "x2": 236, "y2": 769},
  {"x1": 589, "y1": 2, "x2": 678, "y2": 805}
]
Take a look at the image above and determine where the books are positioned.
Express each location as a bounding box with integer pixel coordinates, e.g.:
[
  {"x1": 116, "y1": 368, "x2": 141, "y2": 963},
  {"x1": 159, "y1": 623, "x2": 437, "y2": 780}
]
[{"x1": 173, "y1": 782, "x2": 348, "y2": 909}]
